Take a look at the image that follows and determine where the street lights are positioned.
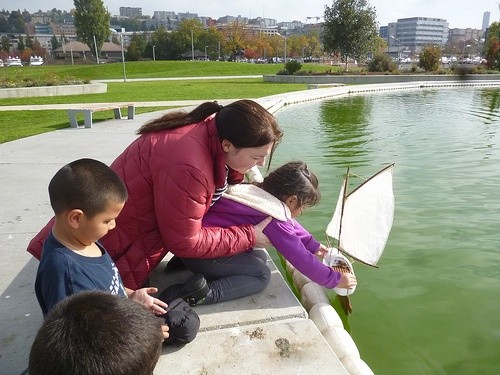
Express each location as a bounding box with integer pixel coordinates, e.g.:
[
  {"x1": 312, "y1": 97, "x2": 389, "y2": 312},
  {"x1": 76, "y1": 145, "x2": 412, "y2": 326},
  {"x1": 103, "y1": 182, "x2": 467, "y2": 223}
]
[
  {"x1": 204, "y1": 46, "x2": 208, "y2": 62},
  {"x1": 153, "y1": 45, "x2": 156, "y2": 61}
]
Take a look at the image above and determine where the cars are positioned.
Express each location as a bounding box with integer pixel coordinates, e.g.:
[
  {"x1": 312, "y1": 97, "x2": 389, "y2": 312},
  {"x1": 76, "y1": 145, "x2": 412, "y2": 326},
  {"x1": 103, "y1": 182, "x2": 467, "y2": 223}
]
[
  {"x1": 233, "y1": 56, "x2": 319, "y2": 63},
  {"x1": 401, "y1": 57, "x2": 420, "y2": 63},
  {"x1": 436, "y1": 56, "x2": 488, "y2": 64}
]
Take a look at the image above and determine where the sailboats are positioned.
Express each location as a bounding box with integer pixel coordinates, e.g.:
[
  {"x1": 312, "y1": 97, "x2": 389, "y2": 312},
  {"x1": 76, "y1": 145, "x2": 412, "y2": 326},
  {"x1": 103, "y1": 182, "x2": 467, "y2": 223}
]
[{"x1": 322, "y1": 161, "x2": 396, "y2": 297}]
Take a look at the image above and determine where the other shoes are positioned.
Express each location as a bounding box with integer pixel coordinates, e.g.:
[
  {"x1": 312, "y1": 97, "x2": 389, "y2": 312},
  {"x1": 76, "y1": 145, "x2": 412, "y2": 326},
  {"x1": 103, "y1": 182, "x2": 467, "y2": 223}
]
[
  {"x1": 159, "y1": 273, "x2": 208, "y2": 305},
  {"x1": 166, "y1": 256, "x2": 192, "y2": 271}
]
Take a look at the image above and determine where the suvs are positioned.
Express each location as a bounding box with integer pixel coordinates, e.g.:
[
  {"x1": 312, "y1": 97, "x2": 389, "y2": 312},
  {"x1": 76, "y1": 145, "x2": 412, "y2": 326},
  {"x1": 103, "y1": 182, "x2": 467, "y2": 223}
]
[
  {"x1": 30, "y1": 56, "x2": 44, "y2": 67},
  {"x1": 7, "y1": 56, "x2": 24, "y2": 67},
  {"x1": 0, "y1": 59, "x2": 4, "y2": 67}
]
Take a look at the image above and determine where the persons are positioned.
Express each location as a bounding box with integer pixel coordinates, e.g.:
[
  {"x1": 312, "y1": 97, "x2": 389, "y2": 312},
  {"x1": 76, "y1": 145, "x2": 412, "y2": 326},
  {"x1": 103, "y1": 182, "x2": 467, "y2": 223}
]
[
  {"x1": 158, "y1": 161, "x2": 357, "y2": 305},
  {"x1": 18, "y1": 291, "x2": 162, "y2": 375},
  {"x1": 27, "y1": 98, "x2": 284, "y2": 291},
  {"x1": 36, "y1": 157, "x2": 169, "y2": 342}
]
